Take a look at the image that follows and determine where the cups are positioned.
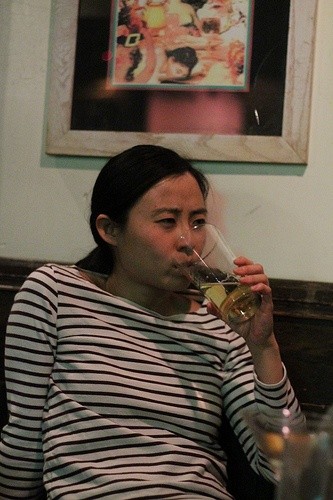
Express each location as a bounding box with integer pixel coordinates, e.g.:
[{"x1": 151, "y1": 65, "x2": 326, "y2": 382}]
[
  {"x1": 173, "y1": 224, "x2": 260, "y2": 326},
  {"x1": 243, "y1": 406, "x2": 333, "y2": 500}
]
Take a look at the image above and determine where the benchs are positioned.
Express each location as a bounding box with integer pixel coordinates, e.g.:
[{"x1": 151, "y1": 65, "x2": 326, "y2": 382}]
[{"x1": 0, "y1": 256, "x2": 333, "y2": 500}]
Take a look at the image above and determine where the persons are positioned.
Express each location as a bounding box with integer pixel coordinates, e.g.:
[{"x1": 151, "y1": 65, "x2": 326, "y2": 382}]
[{"x1": 0, "y1": 144, "x2": 307, "y2": 498}]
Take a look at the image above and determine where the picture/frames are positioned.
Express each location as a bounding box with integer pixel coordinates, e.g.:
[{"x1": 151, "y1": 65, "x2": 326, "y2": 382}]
[{"x1": 44, "y1": 0, "x2": 318, "y2": 164}]
